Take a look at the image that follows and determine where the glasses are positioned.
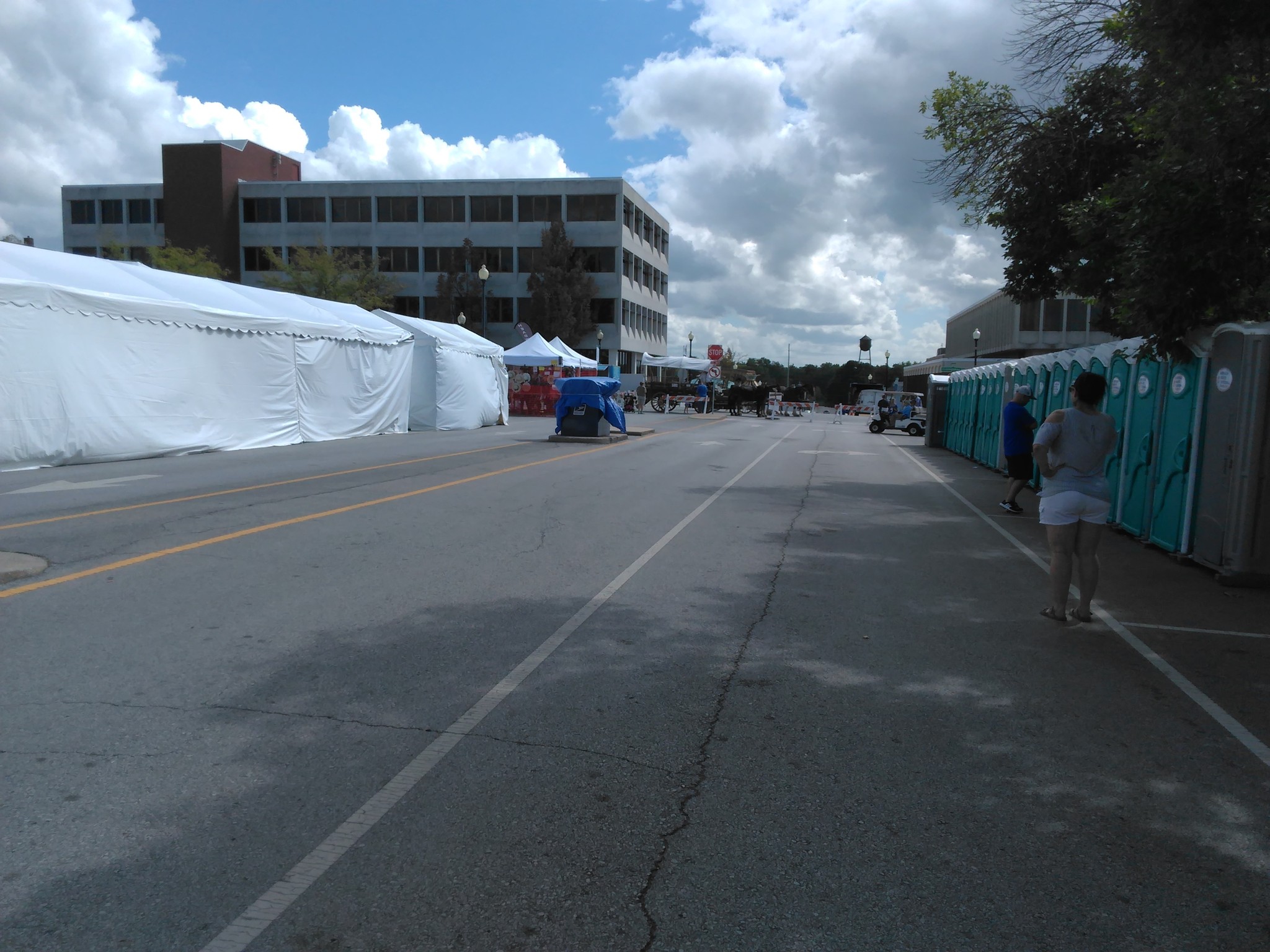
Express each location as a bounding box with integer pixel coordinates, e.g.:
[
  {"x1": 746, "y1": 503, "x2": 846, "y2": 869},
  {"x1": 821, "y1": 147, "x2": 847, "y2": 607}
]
[{"x1": 1070, "y1": 385, "x2": 1076, "y2": 392}]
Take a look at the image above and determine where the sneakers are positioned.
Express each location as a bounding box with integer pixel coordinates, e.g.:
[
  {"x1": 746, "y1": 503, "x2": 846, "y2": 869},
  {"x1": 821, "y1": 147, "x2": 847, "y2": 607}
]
[{"x1": 1000, "y1": 500, "x2": 1023, "y2": 513}]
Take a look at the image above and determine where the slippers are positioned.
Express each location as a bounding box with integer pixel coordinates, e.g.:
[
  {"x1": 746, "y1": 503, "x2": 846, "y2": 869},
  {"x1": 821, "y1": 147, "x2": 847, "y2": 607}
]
[
  {"x1": 1069, "y1": 608, "x2": 1093, "y2": 622},
  {"x1": 1040, "y1": 606, "x2": 1067, "y2": 621}
]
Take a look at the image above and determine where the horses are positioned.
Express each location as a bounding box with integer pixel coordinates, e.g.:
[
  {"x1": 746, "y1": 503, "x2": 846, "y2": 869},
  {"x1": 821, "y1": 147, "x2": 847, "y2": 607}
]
[
  {"x1": 774, "y1": 383, "x2": 815, "y2": 417},
  {"x1": 728, "y1": 382, "x2": 777, "y2": 415}
]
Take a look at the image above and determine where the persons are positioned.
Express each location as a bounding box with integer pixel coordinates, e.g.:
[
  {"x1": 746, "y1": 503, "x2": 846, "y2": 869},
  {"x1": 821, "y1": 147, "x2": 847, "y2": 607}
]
[
  {"x1": 695, "y1": 382, "x2": 708, "y2": 413},
  {"x1": 998, "y1": 386, "x2": 1038, "y2": 513},
  {"x1": 1032, "y1": 371, "x2": 1118, "y2": 622},
  {"x1": 636, "y1": 382, "x2": 647, "y2": 415},
  {"x1": 684, "y1": 394, "x2": 691, "y2": 414},
  {"x1": 876, "y1": 394, "x2": 923, "y2": 426}
]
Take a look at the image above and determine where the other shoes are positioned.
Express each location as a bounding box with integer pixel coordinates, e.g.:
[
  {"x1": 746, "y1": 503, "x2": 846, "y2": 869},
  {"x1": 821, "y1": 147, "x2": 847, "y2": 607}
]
[
  {"x1": 888, "y1": 423, "x2": 895, "y2": 427},
  {"x1": 684, "y1": 412, "x2": 689, "y2": 414},
  {"x1": 638, "y1": 411, "x2": 645, "y2": 414}
]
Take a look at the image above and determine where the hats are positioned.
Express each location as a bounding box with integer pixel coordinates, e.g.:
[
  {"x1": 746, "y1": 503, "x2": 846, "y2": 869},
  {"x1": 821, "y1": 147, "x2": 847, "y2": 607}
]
[
  {"x1": 904, "y1": 400, "x2": 911, "y2": 404},
  {"x1": 1016, "y1": 385, "x2": 1037, "y2": 400}
]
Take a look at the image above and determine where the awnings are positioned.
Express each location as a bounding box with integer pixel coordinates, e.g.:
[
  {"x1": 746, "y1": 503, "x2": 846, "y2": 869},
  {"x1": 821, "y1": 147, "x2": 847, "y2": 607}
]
[{"x1": 902, "y1": 356, "x2": 1019, "y2": 378}]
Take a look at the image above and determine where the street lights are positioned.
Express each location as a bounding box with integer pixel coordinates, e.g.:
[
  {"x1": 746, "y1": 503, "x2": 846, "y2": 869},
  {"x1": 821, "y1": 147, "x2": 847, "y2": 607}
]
[
  {"x1": 971, "y1": 328, "x2": 981, "y2": 368},
  {"x1": 598, "y1": 329, "x2": 603, "y2": 364},
  {"x1": 480, "y1": 263, "x2": 490, "y2": 338},
  {"x1": 884, "y1": 349, "x2": 889, "y2": 389},
  {"x1": 687, "y1": 331, "x2": 694, "y2": 357},
  {"x1": 732, "y1": 362, "x2": 738, "y2": 382}
]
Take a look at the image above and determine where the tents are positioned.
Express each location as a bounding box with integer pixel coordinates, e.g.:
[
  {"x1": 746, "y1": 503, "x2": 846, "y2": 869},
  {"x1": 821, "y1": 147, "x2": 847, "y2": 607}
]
[
  {"x1": 548, "y1": 336, "x2": 599, "y2": 377},
  {"x1": 0, "y1": 239, "x2": 417, "y2": 474},
  {"x1": 503, "y1": 332, "x2": 581, "y2": 417},
  {"x1": 369, "y1": 308, "x2": 509, "y2": 432}
]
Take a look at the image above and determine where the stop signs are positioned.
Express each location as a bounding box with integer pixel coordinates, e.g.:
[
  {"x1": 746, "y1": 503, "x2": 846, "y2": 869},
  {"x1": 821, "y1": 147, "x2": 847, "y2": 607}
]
[{"x1": 708, "y1": 344, "x2": 723, "y2": 361}]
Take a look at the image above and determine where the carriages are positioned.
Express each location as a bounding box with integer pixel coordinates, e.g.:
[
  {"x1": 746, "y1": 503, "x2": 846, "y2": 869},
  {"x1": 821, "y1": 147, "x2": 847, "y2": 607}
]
[{"x1": 640, "y1": 352, "x2": 809, "y2": 425}]
